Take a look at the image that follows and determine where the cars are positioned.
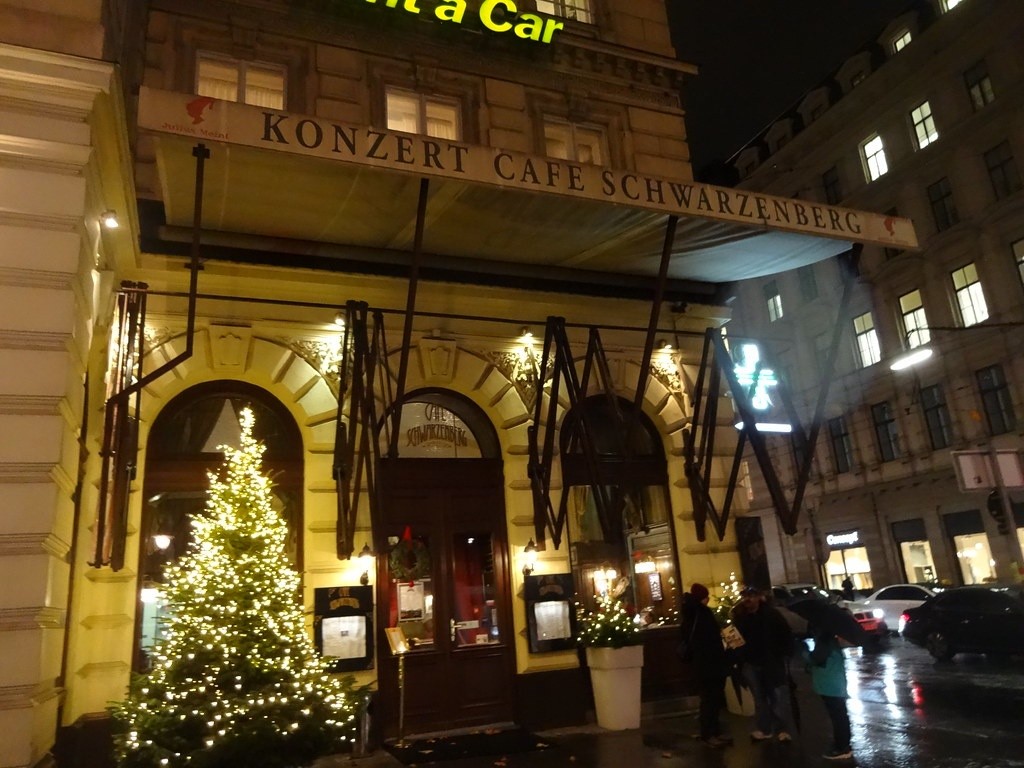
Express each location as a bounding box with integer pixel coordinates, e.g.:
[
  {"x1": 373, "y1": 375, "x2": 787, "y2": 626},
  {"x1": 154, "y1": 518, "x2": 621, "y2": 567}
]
[
  {"x1": 769, "y1": 582, "x2": 854, "y2": 612},
  {"x1": 896, "y1": 583, "x2": 1024, "y2": 662},
  {"x1": 848, "y1": 584, "x2": 937, "y2": 635}
]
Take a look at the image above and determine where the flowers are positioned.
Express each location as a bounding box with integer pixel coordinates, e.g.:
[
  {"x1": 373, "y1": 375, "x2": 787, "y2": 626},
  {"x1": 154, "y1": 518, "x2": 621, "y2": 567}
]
[
  {"x1": 573, "y1": 590, "x2": 651, "y2": 652},
  {"x1": 710, "y1": 571, "x2": 742, "y2": 626}
]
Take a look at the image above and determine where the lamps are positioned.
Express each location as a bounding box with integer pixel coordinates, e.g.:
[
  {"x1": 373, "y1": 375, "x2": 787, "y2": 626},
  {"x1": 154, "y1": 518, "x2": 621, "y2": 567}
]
[
  {"x1": 658, "y1": 338, "x2": 672, "y2": 349},
  {"x1": 99, "y1": 210, "x2": 118, "y2": 228},
  {"x1": 334, "y1": 312, "x2": 346, "y2": 325},
  {"x1": 522, "y1": 537, "x2": 540, "y2": 577},
  {"x1": 357, "y1": 542, "x2": 376, "y2": 584},
  {"x1": 520, "y1": 326, "x2": 536, "y2": 337}
]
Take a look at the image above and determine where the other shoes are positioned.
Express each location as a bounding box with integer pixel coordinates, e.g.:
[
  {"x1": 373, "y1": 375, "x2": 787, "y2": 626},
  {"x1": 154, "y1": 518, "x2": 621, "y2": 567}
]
[
  {"x1": 699, "y1": 734, "x2": 723, "y2": 749},
  {"x1": 750, "y1": 730, "x2": 774, "y2": 741},
  {"x1": 821, "y1": 749, "x2": 851, "y2": 759},
  {"x1": 717, "y1": 734, "x2": 733, "y2": 744},
  {"x1": 778, "y1": 731, "x2": 792, "y2": 744}
]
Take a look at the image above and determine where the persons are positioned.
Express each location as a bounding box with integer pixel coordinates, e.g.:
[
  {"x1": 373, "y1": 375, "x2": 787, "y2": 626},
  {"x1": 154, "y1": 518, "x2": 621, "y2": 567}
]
[
  {"x1": 640, "y1": 606, "x2": 661, "y2": 630},
  {"x1": 809, "y1": 625, "x2": 852, "y2": 758},
  {"x1": 732, "y1": 586, "x2": 796, "y2": 742},
  {"x1": 842, "y1": 577, "x2": 853, "y2": 601},
  {"x1": 680, "y1": 582, "x2": 734, "y2": 747}
]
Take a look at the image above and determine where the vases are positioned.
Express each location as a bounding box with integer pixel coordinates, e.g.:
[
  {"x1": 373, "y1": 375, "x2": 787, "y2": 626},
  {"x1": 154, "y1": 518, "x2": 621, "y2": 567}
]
[
  {"x1": 724, "y1": 677, "x2": 755, "y2": 718},
  {"x1": 583, "y1": 645, "x2": 644, "y2": 731}
]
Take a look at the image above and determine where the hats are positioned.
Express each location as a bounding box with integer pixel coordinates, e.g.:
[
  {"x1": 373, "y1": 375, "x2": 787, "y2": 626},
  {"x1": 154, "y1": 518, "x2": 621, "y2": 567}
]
[{"x1": 691, "y1": 583, "x2": 708, "y2": 601}]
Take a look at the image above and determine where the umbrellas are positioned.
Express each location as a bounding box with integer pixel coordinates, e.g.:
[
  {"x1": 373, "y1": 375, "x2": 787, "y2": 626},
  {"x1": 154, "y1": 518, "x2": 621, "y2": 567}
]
[{"x1": 785, "y1": 600, "x2": 870, "y2": 647}]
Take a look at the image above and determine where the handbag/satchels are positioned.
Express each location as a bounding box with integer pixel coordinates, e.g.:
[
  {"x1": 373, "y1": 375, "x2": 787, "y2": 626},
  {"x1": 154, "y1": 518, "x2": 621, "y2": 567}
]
[{"x1": 677, "y1": 639, "x2": 691, "y2": 662}]
